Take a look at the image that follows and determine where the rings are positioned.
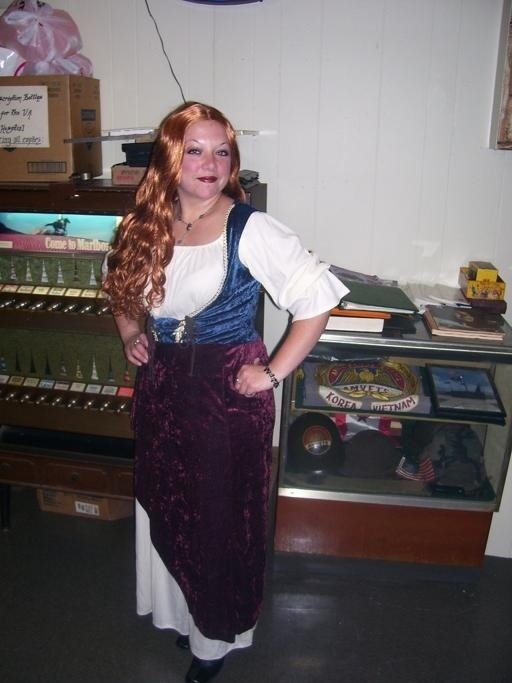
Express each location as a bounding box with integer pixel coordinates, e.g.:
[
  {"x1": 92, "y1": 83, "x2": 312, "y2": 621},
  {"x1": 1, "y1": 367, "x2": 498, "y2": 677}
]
[{"x1": 132, "y1": 339, "x2": 140, "y2": 346}]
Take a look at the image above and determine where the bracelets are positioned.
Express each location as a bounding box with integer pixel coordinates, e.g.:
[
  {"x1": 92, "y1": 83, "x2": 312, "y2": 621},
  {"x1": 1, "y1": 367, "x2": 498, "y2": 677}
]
[{"x1": 262, "y1": 361, "x2": 279, "y2": 389}]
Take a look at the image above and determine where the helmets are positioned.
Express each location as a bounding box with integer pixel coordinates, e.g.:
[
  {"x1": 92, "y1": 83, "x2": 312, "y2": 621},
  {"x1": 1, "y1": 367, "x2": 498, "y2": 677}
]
[{"x1": 286, "y1": 411, "x2": 345, "y2": 472}]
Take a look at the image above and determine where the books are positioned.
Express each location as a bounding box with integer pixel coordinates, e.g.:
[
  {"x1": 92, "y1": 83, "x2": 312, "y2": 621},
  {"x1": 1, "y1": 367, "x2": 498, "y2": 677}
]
[{"x1": 323, "y1": 262, "x2": 508, "y2": 427}]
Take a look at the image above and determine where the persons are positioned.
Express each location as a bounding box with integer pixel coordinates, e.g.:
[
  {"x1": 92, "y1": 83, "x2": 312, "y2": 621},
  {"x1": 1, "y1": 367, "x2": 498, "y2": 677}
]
[{"x1": 101, "y1": 101, "x2": 352, "y2": 682}]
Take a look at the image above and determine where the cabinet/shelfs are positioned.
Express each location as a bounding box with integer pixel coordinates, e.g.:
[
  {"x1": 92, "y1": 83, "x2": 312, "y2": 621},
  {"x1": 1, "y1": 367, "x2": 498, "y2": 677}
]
[
  {"x1": 0, "y1": 180, "x2": 270, "y2": 570},
  {"x1": 272, "y1": 288, "x2": 512, "y2": 574}
]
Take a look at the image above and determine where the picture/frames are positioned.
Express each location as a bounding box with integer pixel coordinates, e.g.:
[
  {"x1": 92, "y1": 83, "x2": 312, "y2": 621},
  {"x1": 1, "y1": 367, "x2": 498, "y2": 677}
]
[
  {"x1": 425, "y1": 362, "x2": 507, "y2": 419},
  {"x1": 489, "y1": 9, "x2": 512, "y2": 154}
]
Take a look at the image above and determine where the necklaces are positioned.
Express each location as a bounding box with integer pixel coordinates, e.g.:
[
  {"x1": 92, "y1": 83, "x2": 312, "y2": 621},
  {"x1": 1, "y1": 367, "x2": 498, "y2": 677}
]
[{"x1": 176, "y1": 210, "x2": 207, "y2": 244}]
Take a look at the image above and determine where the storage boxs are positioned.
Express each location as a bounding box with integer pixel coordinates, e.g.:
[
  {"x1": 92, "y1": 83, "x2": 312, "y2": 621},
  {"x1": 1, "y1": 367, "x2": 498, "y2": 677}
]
[
  {"x1": 0, "y1": 74, "x2": 104, "y2": 182},
  {"x1": 37, "y1": 490, "x2": 135, "y2": 522}
]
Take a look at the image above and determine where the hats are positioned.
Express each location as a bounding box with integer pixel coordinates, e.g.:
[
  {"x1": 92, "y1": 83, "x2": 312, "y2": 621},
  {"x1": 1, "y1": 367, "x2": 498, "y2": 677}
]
[{"x1": 338, "y1": 429, "x2": 418, "y2": 478}]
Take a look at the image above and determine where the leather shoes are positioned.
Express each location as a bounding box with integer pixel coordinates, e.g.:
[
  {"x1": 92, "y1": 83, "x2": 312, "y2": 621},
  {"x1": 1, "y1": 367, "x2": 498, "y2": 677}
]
[
  {"x1": 176, "y1": 634, "x2": 190, "y2": 648},
  {"x1": 185, "y1": 654, "x2": 225, "y2": 682}
]
